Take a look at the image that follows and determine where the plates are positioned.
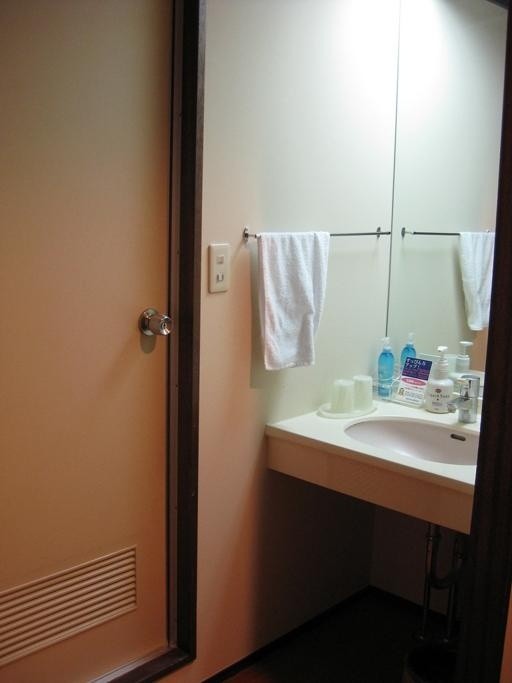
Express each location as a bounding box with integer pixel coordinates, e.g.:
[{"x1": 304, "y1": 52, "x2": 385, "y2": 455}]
[{"x1": 319, "y1": 400, "x2": 380, "y2": 420}]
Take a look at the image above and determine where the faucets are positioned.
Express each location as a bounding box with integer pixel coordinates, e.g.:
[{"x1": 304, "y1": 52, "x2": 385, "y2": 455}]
[{"x1": 448, "y1": 374, "x2": 480, "y2": 423}]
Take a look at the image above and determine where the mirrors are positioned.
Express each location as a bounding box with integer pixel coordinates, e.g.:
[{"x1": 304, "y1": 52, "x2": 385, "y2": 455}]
[{"x1": 385, "y1": 0, "x2": 508, "y2": 405}]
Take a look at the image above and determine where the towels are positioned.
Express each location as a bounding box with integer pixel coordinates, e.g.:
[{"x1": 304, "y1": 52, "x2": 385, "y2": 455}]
[
  {"x1": 257, "y1": 231, "x2": 330, "y2": 372},
  {"x1": 459, "y1": 230, "x2": 496, "y2": 334}
]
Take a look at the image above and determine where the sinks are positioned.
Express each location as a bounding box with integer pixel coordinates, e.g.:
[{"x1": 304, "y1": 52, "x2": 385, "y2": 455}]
[{"x1": 344, "y1": 414, "x2": 479, "y2": 471}]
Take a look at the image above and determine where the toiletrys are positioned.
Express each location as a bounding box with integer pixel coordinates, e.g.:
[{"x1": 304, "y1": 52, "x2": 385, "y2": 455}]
[
  {"x1": 400, "y1": 332, "x2": 417, "y2": 373},
  {"x1": 377, "y1": 336, "x2": 394, "y2": 397}
]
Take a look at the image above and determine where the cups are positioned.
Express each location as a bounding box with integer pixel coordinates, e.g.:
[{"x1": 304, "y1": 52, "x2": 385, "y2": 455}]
[
  {"x1": 354, "y1": 375, "x2": 373, "y2": 408},
  {"x1": 332, "y1": 380, "x2": 354, "y2": 412}
]
